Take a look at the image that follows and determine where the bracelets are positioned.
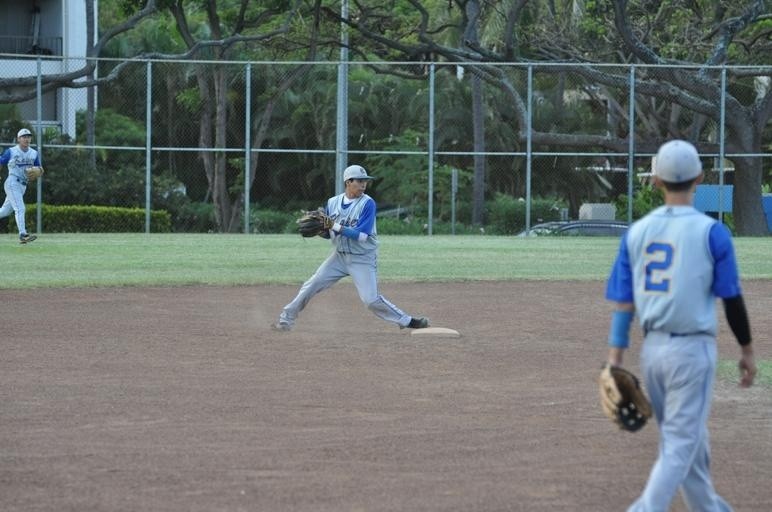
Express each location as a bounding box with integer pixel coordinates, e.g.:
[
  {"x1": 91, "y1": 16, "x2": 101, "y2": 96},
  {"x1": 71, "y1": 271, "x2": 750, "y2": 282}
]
[{"x1": 330, "y1": 222, "x2": 342, "y2": 232}]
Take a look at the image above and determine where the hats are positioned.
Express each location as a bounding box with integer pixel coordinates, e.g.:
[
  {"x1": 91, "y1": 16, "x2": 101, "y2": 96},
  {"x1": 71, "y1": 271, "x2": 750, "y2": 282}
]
[
  {"x1": 16, "y1": 127, "x2": 33, "y2": 140},
  {"x1": 344, "y1": 164, "x2": 376, "y2": 185},
  {"x1": 651, "y1": 138, "x2": 704, "y2": 185}
]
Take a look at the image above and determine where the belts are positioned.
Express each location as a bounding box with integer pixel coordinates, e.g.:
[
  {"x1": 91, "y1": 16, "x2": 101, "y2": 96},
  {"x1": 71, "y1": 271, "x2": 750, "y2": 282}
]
[{"x1": 639, "y1": 329, "x2": 716, "y2": 338}]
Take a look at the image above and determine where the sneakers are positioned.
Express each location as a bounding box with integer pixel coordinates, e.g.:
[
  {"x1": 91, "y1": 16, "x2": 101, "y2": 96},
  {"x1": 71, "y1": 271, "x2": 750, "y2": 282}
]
[
  {"x1": 18, "y1": 233, "x2": 37, "y2": 245},
  {"x1": 408, "y1": 316, "x2": 429, "y2": 329}
]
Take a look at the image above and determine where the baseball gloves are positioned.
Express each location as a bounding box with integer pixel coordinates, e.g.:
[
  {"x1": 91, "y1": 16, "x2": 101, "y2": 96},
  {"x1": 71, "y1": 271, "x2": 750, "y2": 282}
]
[
  {"x1": 26, "y1": 167, "x2": 44, "y2": 182},
  {"x1": 599, "y1": 366, "x2": 652, "y2": 431},
  {"x1": 297, "y1": 209, "x2": 331, "y2": 237}
]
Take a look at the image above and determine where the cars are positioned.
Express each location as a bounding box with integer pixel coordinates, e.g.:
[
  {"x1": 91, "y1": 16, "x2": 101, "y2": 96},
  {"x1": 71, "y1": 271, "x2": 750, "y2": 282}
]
[{"x1": 519, "y1": 221, "x2": 628, "y2": 238}]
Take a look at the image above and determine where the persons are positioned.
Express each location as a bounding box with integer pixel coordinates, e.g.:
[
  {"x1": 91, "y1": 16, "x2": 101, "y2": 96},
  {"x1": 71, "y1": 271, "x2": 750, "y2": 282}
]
[
  {"x1": 605, "y1": 139, "x2": 759, "y2": 512},
  {"x1": 0, "y1": 129, "x2": 44, "y2": 244},
  {"x1": 275, "y1": 163, "x2": 430, "y2": 332}
]
